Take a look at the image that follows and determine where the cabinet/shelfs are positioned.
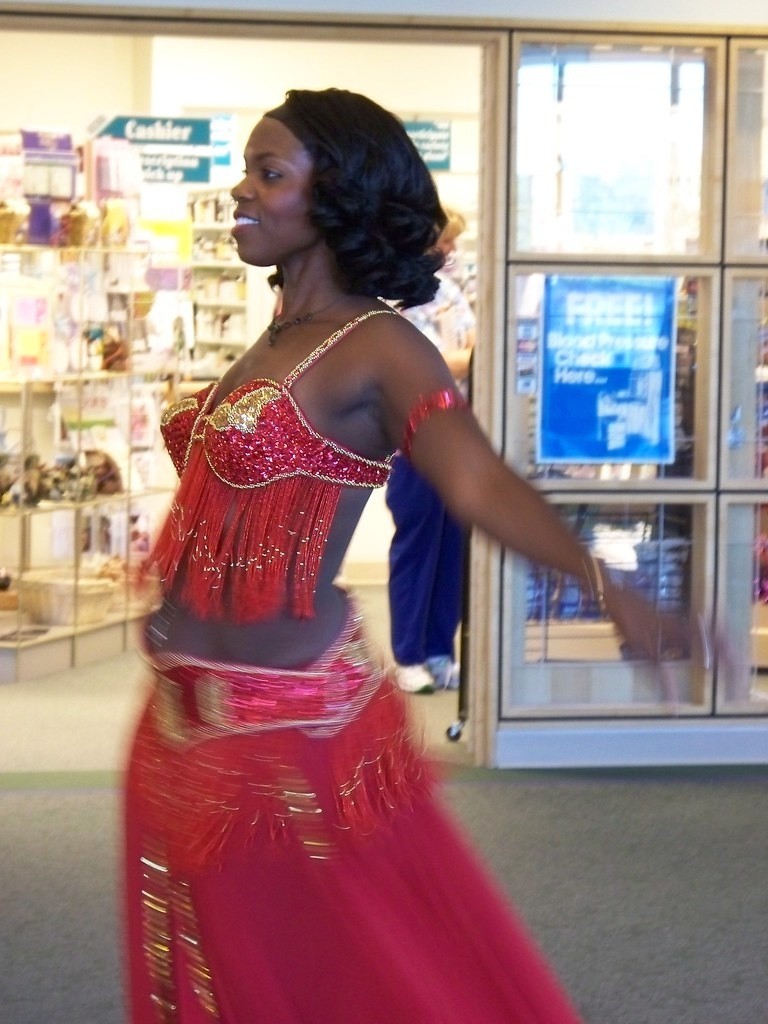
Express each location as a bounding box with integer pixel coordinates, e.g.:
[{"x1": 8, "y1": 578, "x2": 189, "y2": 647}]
[{"x1": 1, "y1": 188, "x2": 280, "y2": 690}]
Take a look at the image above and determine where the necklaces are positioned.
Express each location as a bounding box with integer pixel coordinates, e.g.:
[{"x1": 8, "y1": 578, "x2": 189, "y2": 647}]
[{"x1": 266, "y1": 297, "x2": 345, "y2": 345}]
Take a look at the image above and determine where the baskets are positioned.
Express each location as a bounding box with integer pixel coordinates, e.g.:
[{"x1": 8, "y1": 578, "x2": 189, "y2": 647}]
[{"x1": 20, "y1": 569, "x2": 115, "y2": 625}]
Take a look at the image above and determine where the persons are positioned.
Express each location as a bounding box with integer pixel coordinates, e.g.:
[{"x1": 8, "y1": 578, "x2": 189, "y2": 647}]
[
  {"x1": 114, "y1": 87, "x2": 727, "y2": 1023},
  {"x1": 384, "y1": 206, "x2": 477, "y2": 692}
]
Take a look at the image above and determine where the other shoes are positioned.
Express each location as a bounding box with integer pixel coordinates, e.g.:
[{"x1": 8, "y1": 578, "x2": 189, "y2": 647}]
[
  {"x1": 426, "y1": 654, "x2": 460, "y2": 689},
  {"x1": 395, "y1": 665, "x2": 435, "y2": 693}
]
